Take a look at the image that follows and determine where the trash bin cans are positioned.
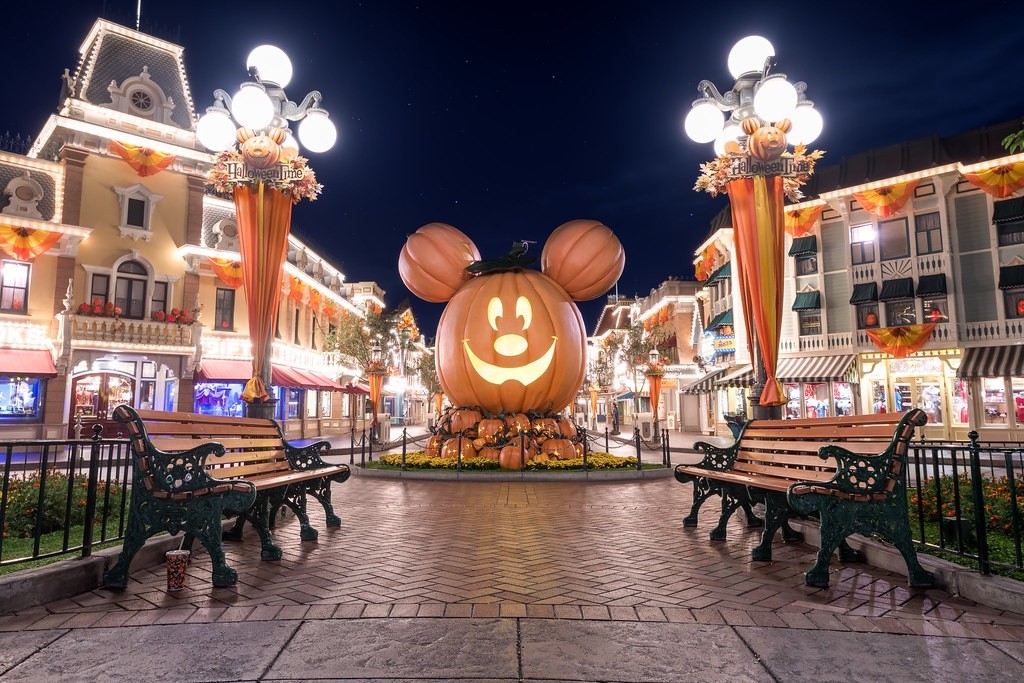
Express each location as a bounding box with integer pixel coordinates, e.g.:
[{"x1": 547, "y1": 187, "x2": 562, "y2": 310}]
[
  {"x1": 574, "y1": 413, "x2": 584, "y2": 427},
  {"x1": 588, "y1": 413, "x2": 597, "y2": 430},
  {"x1": 631, "y1": 412, "x2": 653, "y2": 441},
  {"x1": 667, "y1": 411, "x2": 679, "y2": 430},
  {"x1": 425, "y1": 413, "x2": 437, "y2": 431},
  {"x1": 369, "y1": 413, "x2": 391, "y2": 444}
]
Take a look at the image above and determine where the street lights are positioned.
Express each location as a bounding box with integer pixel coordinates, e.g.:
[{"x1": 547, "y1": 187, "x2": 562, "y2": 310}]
[
  {"x1": 683, "y1": 34, "x2": 828, "y2": 420},
  {"x1": 195, "y1": 44, "x2": 341, "y2": 417}
]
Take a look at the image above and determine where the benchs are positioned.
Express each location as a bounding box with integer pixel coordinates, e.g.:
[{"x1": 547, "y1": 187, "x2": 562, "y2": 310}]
[
  {"x1": 99, "y1": 405, "x2": 350, "y2": 594},
  {"x1": 674, "y1": 408, "x2": 947, "y2": 591}
]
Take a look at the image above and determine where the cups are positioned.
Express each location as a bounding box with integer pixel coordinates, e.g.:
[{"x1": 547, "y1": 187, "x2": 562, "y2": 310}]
[{"x1": 166, "y1": 550, "x2": 190, "y2": 590}]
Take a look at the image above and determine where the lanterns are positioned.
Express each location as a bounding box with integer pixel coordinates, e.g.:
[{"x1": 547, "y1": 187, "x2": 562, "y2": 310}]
[{"x1": 398, "y1": 220, "x2": 627, "y2": 416}]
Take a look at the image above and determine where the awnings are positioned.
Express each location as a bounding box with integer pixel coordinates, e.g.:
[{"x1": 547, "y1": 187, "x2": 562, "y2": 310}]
[
  {"x1": 849, "y1": 273, "x2": 946, "y2": 306},
  {"x1": 998, "y1": 264, "x2": 1024, "y2": 290},
  {"x1": 991, "y1": 195, "x2": 1024, "y2": 224},
  {"x1": 956, "y1": 346, "x2": 1024, "y2": 379},
  {"x1": 792, "y1": 290, "x2": 821, "y2": 311},
  {"x1": 705, "y1": 308, "x2": 733, "y2": 331},
  {"x1": 676, "y1": 363, "x2": 755, "y2": 395},
  {"x1": 607, "y1": 392, "x2": 634, "y2": 400},
  {"x1": 774, "y1": 354, "x2": 860, "y2": 385},
  {"x1": 702, "y1": 260, "x2": 731, "y2": 287},
  {"x1": 789, "y1": 235, "x2": 817, "y2": 257}
]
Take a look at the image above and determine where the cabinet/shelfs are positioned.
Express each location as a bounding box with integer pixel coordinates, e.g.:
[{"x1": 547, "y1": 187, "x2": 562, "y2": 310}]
[{"x1": 875, "y1": 375, "x2": 1024, "y2": 423}]
